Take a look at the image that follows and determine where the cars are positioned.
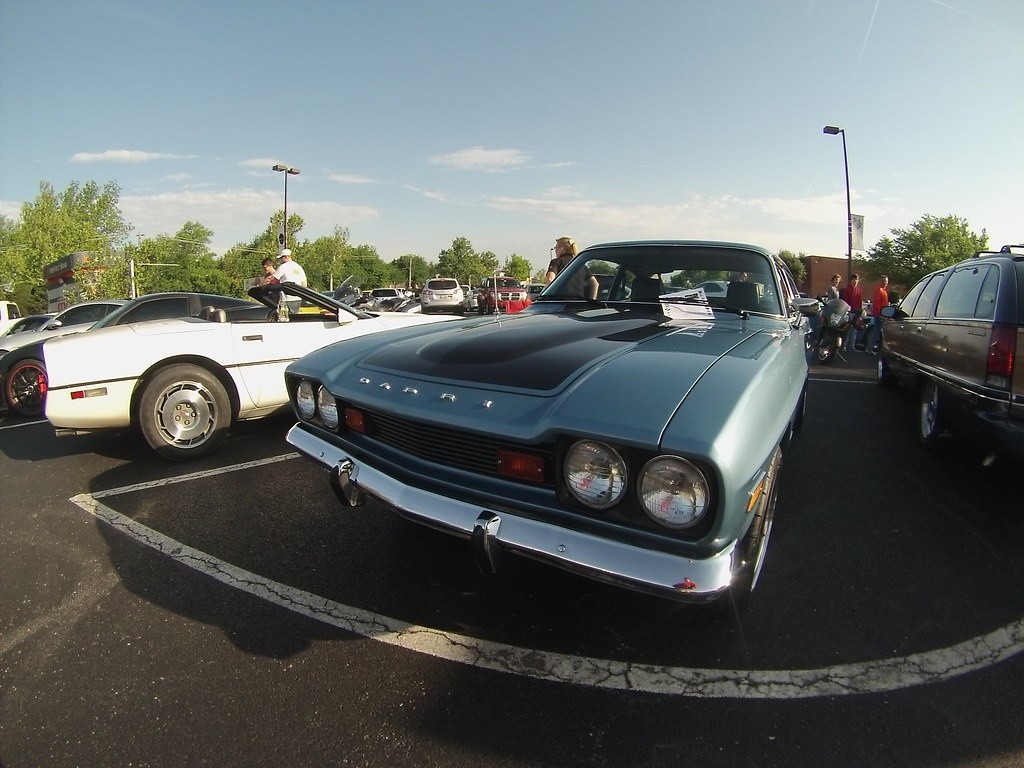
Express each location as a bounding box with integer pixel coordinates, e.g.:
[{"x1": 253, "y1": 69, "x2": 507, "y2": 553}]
[
  {"x1": 39, "y1": 282, "x2": 469, "y2": 463},
  {"x1": 0, "y1": 300, "x2": 59, "y2": 342},
  {"x1": 460, "y1": 285, "x2": 479, "y2": 312},
  {"x1": 692, "y1": 280, "x2": 730, "y2": 298},
  {"x1": 295, "y1": 274, "x2": 422, "y2": 314},
  {"x1": 526, "y1": 283, "x2": 546, "y2": 301},
  {"x1": 284, "y1": 241, "x2": 822, "y2": 614},
  {"x1": 0, "y1": 293, "x2": 262, "y2": 421}
]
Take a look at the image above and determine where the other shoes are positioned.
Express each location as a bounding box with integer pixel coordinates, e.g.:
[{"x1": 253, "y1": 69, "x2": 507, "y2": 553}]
[
  {"x1": 843, "y1": 347, "x2": 859, "y2": 352},
  {"x1": 865, "y1": 349, "x2": 878, "y2": 355}
]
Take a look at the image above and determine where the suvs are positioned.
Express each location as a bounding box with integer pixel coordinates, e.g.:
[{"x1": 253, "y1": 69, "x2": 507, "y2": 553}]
[
  {"x1": 477, "y1": 276, "x2": 527, "y2": 316},
  {"x1": 591, "y1": 272, "x2": 615, "y2": 301},
  {"x1": 420, "y1": 277, "x2": 464, "y2": 315},
  {"x1": 876, "y1": 246, "x2": 1024, "y2": 453}
]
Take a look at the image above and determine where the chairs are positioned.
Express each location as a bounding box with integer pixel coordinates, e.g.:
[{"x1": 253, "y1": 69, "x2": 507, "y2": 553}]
[
  {"x1": 724, "y1": 282, "x2": 761, "y2": 311},
  {"x1": 213, "y1": 309, "x2": 227, "y2": 323},
  {"x1": 630, "y1": 278, "x2": 665, "y2": 314},
  {"x1": 200, "y1": 305, "x2": 215, "y2": 322}
]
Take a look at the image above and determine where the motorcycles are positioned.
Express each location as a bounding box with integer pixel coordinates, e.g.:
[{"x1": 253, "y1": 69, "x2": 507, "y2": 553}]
[{"x1": 817, "y1": 295, "x2": 849, "y2": 364}]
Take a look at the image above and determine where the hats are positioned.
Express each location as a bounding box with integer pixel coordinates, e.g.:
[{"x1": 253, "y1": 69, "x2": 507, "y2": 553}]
[{"x1": 276, "y1": 249, "x2": 292, "y2": 258}]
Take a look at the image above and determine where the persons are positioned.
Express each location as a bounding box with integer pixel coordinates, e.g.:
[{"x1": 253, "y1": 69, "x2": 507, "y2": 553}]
[
  {"x1": 842, "y1": 273, "x2": 863, "y2": 351},
  {"x1": 865, "y1": 274, "x2": 891, "y2": 356},
  {"x1": 730, "y1": 271, "x2": 748, "y2": 283},
  {"x1": 546, "y1": 236, "x2": 599, "y2": 302},
  {"x1": 257, "y1": 248, "x2": 308, "y2": 301},
  {"x1": 826, "y1": 274, "x2": 842, "y2": 300},
  {"x1": 261, "y1": 257, "x2": 281, "y2": 286}
]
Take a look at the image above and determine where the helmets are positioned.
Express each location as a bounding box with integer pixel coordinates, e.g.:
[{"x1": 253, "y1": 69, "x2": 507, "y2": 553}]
[{"x1": 851, "y1": 315, "x2": 869, "y2": 331}]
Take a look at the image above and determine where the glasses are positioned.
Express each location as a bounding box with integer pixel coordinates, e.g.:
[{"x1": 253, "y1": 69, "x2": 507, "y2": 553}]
[{"x1": 554, "y1": 247, "x2": 557, "y2": 249}]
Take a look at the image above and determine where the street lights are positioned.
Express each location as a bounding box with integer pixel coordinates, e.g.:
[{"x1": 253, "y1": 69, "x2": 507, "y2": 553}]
[
  {"x1": 822, "y1": 125, "x2": 852, "y2": 286},
  {"x1": 271, "y1": 164, "x2": 301, "y2": 248}
]
